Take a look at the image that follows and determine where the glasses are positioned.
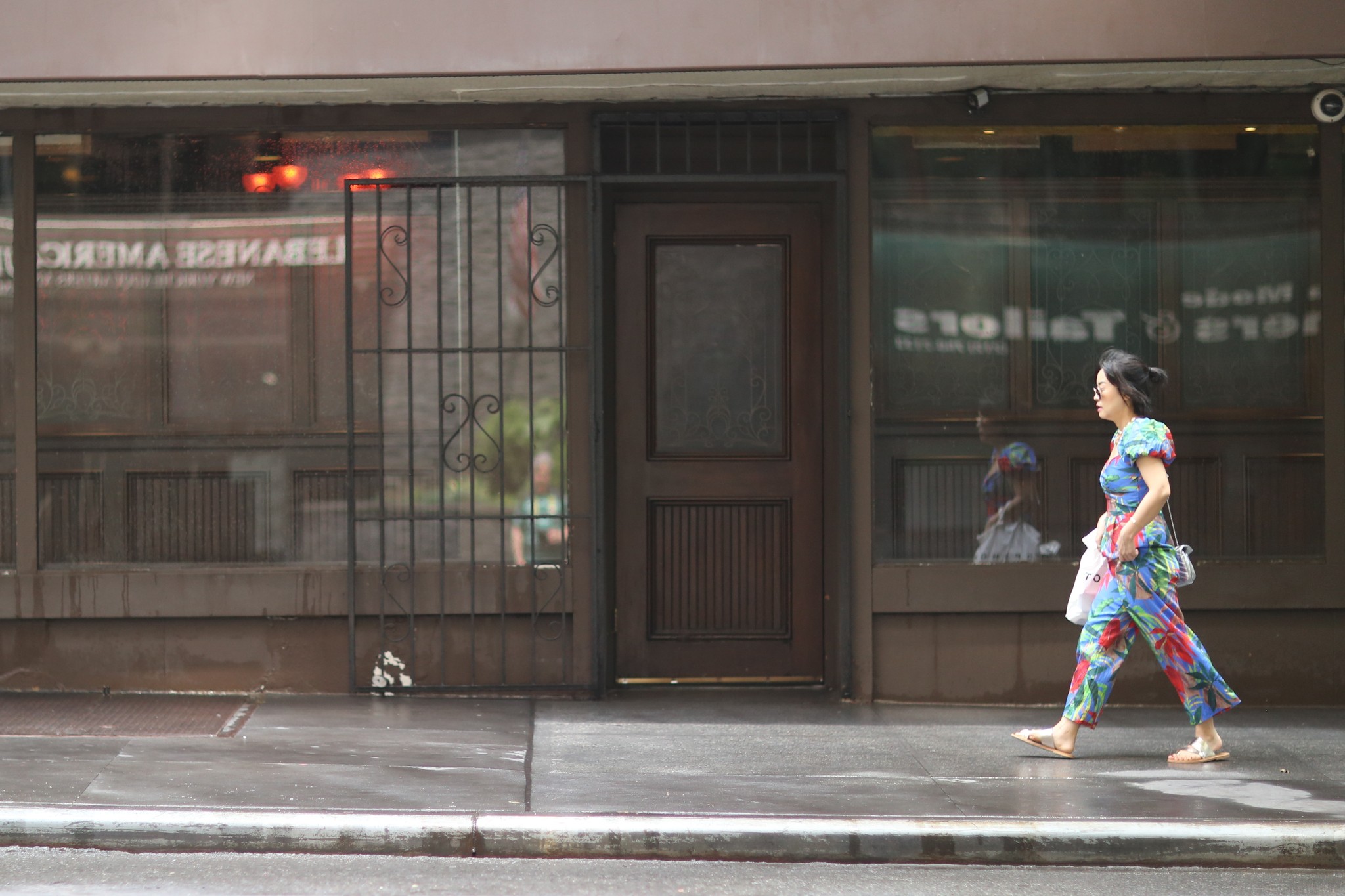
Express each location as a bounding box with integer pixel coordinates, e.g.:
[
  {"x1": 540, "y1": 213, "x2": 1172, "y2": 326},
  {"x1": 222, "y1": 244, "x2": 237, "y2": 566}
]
[{"x1": 1092, "y1": 387, "x2": 1110, "y2": 399}]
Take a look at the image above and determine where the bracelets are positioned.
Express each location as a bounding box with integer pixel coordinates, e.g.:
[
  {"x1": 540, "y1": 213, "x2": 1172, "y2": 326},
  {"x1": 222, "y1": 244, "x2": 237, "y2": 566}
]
[{"x1": 1130, "y1": 517, "x2": 1141, "y2": 529}]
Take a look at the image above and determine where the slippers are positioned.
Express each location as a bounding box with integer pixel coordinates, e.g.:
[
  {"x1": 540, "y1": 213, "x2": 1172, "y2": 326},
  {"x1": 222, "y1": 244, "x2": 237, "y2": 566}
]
[
  {"x1": 1010, "y1": 727, "x2": 1075, "y2": 760},
  {"x1": 1167, "y1": 736, "x2": 1231, "y2": 764}
]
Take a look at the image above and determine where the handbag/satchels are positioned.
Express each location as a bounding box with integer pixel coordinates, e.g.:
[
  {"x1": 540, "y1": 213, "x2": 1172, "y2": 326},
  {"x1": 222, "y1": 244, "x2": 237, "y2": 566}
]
[
  {"x1": 1040, "y1": 540, "x2": 1062, "y2": 560},
  {"x1": 972, "y1": 502, "x2": 1040, "y2": 568},
  {"x1": 1173, "y1": 544, "x2": 1195, "y2": 588},
  {"x1": 1065, "y1": 524, "x2": 1115, "y2": 626}
]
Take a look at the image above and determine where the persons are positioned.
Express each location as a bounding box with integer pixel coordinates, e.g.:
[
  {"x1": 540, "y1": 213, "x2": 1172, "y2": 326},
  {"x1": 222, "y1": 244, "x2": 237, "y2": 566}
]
[
  {"x1": 508, "y1": 451, "x2": 568, "y2": 567},
  {"x1": 973, "y1": 399, "x2": 1048, "y2": 564},
  {"x1": 1010, "y1": 348, "x2": 1241, "y2": 765}
]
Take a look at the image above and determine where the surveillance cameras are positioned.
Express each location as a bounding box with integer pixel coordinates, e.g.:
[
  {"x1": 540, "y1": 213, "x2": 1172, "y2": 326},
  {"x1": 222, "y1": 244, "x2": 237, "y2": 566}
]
[{"x1": 1309, "y1": 89, "x2": 1345, "y2": 124}]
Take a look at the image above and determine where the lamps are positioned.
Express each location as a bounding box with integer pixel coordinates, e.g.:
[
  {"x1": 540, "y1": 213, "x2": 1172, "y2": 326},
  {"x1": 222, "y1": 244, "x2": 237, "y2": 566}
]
[
  {"x1": 968, "y1": 85, "x2": 990, "y2": 111},
  {"x1": 240, "y1": 131, "x2": 308, "y2": 196}
]
